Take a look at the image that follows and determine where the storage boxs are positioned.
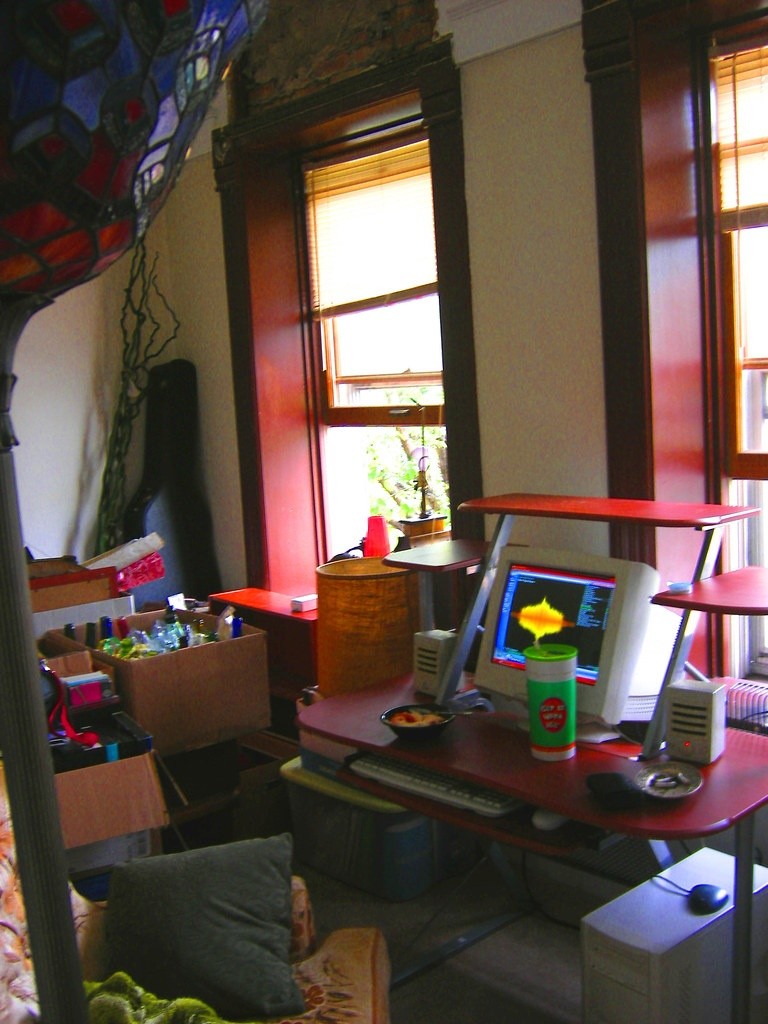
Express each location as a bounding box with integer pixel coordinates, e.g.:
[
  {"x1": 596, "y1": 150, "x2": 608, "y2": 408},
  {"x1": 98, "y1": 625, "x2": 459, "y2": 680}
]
[
  {"x1": 35, "y1": 633, "x2": 92, "y2": 677},
  {"x1": 44, "y1": 609, "x2": 272, "y2": 754},
  {"x1": 51, "y1": 749, "x2": 190, "y2": 904}
]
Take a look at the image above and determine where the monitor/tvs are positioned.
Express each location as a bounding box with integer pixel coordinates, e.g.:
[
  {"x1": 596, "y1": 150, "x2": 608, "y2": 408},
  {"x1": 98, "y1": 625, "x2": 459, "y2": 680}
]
[{"x1": 474, "y1": 545, "x2": 695, "y2": 743}]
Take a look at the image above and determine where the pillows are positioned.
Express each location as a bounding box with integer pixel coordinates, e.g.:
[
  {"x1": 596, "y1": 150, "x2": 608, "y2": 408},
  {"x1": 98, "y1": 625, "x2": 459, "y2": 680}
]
[{"x1": 98, "y1": 833, "x2": 305, "y2": 1020}]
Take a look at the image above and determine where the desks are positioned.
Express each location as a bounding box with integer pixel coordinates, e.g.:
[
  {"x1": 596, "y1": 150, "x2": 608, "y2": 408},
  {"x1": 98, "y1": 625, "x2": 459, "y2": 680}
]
[{"x1": 297, "y1": 670, "x2": 766, "y2": 1023}]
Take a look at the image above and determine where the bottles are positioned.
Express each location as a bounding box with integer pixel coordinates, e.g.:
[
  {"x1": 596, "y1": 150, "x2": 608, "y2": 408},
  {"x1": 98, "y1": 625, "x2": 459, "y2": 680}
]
[{"x1": 64, "y1": 604, "x2": 244, "y2": 648}]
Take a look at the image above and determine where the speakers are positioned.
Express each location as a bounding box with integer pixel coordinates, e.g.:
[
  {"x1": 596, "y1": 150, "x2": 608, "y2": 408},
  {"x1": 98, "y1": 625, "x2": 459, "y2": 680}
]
[
  {"x1": 415, "y1": 630, "x2": 465, "y2": 697},
  {"x1": 664, "y1": 680, "x2": 727, "y2": 764}
]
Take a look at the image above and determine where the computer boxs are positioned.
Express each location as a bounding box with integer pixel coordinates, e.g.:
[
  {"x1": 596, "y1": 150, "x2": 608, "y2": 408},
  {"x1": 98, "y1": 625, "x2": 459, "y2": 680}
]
[{"x1": 581, "y1": 847, "x2": 768, "y2": 1024}]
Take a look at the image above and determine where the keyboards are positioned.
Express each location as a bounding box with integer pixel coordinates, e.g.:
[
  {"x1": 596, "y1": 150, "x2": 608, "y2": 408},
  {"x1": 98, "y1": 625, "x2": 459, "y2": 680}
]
[{"x1": 349, "y1": 751, "x2": 527, "y2": 817}]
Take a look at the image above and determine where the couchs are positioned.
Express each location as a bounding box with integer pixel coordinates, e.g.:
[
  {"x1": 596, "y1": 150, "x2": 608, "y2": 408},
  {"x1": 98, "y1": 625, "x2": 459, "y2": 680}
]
[{"x1": 0, "y1": 764, "x2": 392, "y2": 1024}]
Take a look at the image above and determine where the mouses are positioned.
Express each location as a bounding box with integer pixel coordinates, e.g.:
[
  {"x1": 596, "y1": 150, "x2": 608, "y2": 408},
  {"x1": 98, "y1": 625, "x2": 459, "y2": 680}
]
[
  {"x1": 533, "y1": 809, "x2": 571, "y2": 830},
  {"x1": 689, "y1": 884, "x2": 728, "y2": 914}
]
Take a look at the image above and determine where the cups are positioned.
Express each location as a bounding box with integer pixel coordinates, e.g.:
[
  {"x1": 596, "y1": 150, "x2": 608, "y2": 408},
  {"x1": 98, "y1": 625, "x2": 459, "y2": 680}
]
[{"x1": 524, "y1": 644, "x2": 578, "y2": 762}]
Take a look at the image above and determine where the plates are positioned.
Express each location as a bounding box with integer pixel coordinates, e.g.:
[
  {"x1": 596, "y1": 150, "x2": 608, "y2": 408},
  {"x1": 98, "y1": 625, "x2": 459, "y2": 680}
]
[{"x1": 635, "y1": 762, "x2": 703, "y2": 798}]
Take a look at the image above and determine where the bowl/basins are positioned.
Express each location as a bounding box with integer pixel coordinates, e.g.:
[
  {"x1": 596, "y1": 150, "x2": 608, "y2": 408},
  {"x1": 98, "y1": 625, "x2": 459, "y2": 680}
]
[{"x1": 380, "y1": 704, "x2": 455, "y2": 736}]
